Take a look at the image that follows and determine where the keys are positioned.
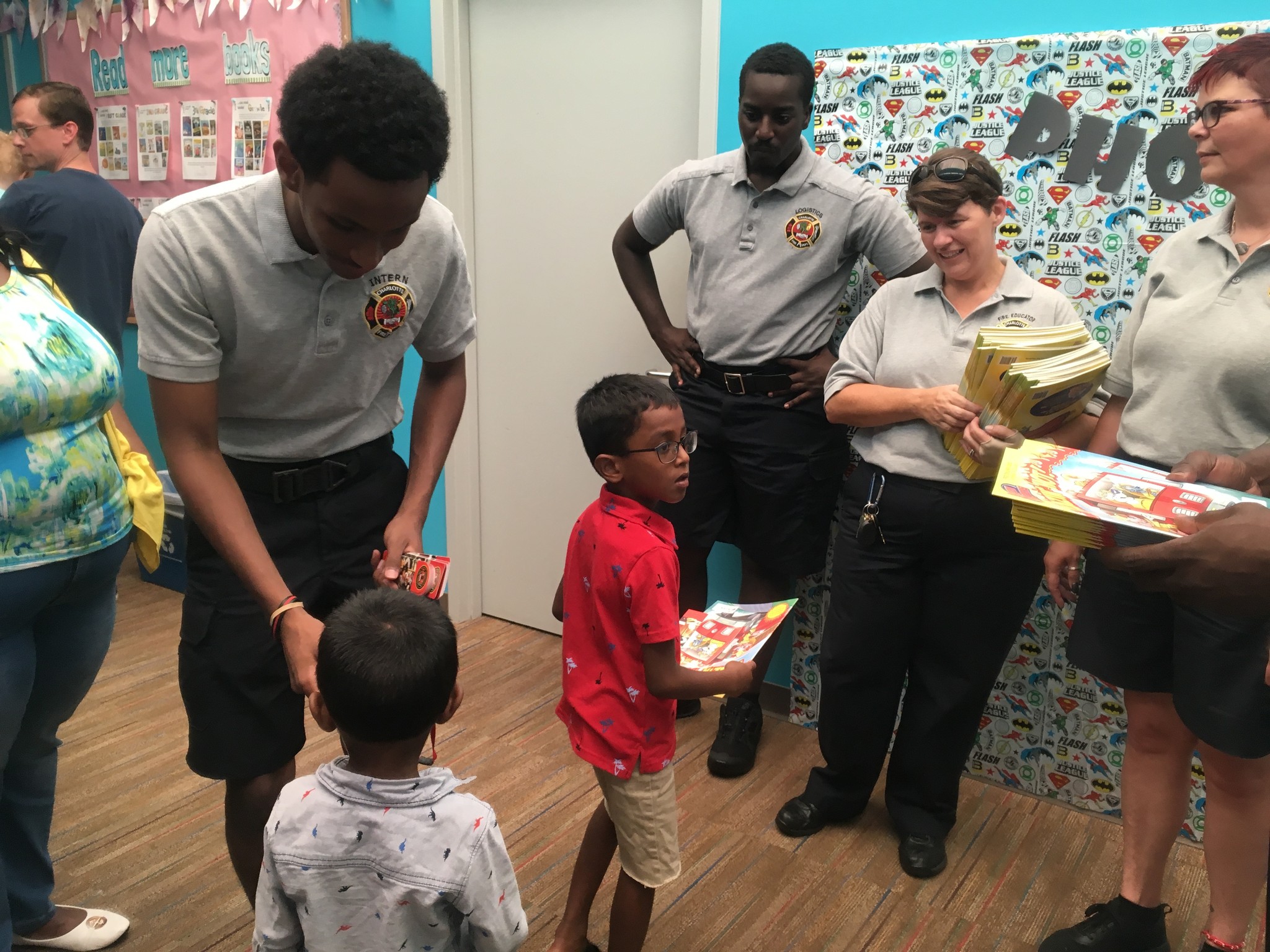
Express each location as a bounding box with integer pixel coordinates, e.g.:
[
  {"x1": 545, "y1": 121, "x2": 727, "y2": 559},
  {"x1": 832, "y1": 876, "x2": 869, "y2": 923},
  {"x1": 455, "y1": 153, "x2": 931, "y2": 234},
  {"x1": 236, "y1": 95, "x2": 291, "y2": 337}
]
[{"x1": 856, "y1": 511, "x2": 886, "y2": 544}]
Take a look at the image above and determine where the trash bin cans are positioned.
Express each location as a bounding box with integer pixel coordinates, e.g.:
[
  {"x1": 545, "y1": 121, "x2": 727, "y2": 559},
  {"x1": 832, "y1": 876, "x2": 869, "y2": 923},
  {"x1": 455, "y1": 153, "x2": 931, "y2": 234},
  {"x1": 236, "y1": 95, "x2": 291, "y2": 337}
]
[{"x1": 135, "y1": 469, "x2": 188, "y2": 594}]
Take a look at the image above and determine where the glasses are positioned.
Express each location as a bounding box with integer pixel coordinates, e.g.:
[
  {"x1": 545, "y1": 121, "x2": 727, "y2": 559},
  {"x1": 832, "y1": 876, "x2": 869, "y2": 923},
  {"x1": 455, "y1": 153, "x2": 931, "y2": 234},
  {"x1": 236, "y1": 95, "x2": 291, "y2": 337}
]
[
  {"x1": 1187, "y1": 98, "x2": 1270, "y2": 131},
  {"x1": 8, "y1": 124, "x2": 51, "y2": 141},
  {"x1": 618, "y1": 429, "x2": 699, "y2": 465},
  {"x1": 907, "y1": 156, "x2": 1002, "y2": 196}
]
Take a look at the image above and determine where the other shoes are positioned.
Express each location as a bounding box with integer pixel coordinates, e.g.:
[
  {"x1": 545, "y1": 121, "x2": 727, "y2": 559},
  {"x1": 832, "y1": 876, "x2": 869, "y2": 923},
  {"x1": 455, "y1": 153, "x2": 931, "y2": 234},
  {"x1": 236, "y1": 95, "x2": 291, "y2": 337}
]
[{"x1": 11, "y1": 903, "x2": 130, "y2": 952}]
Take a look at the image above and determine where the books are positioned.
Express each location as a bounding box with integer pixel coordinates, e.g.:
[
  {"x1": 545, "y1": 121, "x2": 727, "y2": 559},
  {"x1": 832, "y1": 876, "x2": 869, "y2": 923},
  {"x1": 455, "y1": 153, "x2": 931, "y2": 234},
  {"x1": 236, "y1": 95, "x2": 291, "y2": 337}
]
[
  {"x1": 992, "y1": 440, "x2": 1270, "y2": 558},
  {"x1": 944, "y1": 319, "x2": 1114, "y2": 480},
  {"x1": 675, "y1": 594, "x2": 799, "y2": 703}
]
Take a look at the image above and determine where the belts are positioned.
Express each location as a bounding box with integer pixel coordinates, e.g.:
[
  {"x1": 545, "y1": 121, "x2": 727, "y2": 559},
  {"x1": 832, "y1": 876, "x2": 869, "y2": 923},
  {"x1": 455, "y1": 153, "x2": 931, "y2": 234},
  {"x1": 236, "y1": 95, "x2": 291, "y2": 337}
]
[
  {"x1": 690, "y1": 350, "x2": 793, "y2": 396},
  {"x1": 225, "y1": 430, "x2": 393, "y2": 506}
]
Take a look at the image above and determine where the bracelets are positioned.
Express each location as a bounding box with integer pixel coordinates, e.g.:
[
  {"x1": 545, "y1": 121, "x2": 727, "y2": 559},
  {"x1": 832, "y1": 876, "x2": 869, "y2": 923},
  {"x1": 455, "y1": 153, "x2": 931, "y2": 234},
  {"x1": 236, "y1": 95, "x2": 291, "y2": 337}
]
[{"x1": 269, "y1": 595, "x2": 304, "y2": 641}]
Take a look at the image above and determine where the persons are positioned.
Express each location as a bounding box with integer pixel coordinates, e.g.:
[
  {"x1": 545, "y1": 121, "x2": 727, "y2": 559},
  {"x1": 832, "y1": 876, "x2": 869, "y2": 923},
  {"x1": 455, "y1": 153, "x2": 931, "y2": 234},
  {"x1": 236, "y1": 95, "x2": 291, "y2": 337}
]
[
  {"x1": 608, "y1": 43, "x2": 936, "y2": 779},
  {"x1": 546, "y1": 373, "x2": 759, "y2": 952},
  {"x1": 0, "y1": 82, "x2": 145, "y2": 475},
  {"x1": 250, "y1": 588, "x2": 529, "y2": 952},
  {"x1": 775, "y1": 147, "x2": 1102, "y2": 881},
  {"x1": 131, "y1": 43, "x2": 475, "y2": 909},
  {"x1": 1037, "y1": 32, "x2": 1270, "y2": 952},
  {"x1": 0, "y1": 230, "x2": 157, "y2": 952}
]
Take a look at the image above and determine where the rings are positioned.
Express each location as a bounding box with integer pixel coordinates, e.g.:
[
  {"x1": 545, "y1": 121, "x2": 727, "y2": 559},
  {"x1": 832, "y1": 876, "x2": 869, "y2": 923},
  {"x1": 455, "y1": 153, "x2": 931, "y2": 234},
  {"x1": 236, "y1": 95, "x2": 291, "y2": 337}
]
[
  {"x1": 1068, "y1": 567, "x2": 1080, "y2": 570},
  {"x1": 968, "y1": 449, "x2": 974, "y2": 456}
]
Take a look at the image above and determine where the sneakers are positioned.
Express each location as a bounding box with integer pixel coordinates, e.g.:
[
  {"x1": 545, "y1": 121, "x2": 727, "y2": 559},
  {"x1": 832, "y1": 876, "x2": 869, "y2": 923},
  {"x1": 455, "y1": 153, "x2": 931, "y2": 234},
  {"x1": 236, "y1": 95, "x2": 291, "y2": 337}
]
[
  {"x1": 707, "y1": 694, "x2": 764, "y2": 779},
  {"x1": 675, "y1": 696, "x2": 702, "y2": 720},
  {"x1": 898, "y1": 828, "x2": 947, "y2": 880},
  {"x1": 774, "y1": 792, "x2": 840, "y2": 838},
  {"x1": 1037, "y1": 900, "x2": 1173, "y2": 952}
]
